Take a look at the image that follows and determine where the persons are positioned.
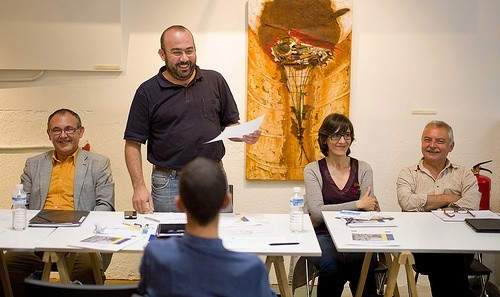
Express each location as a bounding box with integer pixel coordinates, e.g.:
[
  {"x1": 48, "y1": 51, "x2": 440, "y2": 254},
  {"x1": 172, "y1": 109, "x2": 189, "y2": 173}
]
[
  {"x1": 396, "y1": 121, "x2": 481, "y2": 297},
  {"x1": 0, "y1": 109, "x2": 115, "y2": 297},
  {"x1": 123, "y1": 25, "x2": 261, "y2": 213},
  {"x1": 303, "y1": 114, "x2": 382, "y2": 297},
  {"x1": 137, "y1": 157, "x2": 279, "y2": 297}
]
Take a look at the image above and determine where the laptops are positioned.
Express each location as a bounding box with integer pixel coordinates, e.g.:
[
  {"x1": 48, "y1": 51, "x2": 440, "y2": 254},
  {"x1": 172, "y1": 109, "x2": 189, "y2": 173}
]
[{"x1": 466, "y1": 219, "x2": 500, "y2": 233}]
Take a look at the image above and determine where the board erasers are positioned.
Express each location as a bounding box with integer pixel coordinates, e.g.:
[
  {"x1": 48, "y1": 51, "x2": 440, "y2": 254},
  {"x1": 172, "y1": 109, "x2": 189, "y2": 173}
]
[{"x1": 95, "y1": 65, "x2": 120, "y2": 70}]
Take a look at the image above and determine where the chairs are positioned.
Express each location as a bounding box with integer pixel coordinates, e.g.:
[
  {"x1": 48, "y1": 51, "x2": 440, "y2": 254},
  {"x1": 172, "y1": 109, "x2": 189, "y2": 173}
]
[{"x1": 24, "y1": 185, "x2": 500, "y2": 297}]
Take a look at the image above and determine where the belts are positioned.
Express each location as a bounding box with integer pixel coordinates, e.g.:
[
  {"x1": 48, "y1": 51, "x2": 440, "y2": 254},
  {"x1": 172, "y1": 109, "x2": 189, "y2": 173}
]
[{"x1": 154, "y1": 165, "x2": 182, "y2": 177}]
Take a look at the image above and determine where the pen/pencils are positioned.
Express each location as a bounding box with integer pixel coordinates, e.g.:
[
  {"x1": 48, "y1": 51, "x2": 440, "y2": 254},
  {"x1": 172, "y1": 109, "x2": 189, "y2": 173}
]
[
  {"x1": 346, "y1": 217, "x2": 353, "y2": 225},
  {"x1": 270, "y1": 243, "x2": 300, "y2": 246},
  {"x1": 144, "y1": 216, "x2": 159, "y2": 223},
  {"x1": 38, "y1": 215, "x2": 52, "y2": 222}
]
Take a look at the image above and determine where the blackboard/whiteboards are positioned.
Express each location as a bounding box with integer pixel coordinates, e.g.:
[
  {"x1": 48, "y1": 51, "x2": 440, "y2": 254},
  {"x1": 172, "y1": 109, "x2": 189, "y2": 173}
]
[{"x1": 0, "y1": 0, "x2": 122, "y2": 72}]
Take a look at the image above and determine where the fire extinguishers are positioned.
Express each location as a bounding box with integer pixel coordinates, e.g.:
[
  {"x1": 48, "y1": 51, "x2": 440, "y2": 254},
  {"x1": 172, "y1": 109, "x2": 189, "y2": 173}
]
[{"x1": 471, "y1": 160, "x2": 493, "y2": 211}]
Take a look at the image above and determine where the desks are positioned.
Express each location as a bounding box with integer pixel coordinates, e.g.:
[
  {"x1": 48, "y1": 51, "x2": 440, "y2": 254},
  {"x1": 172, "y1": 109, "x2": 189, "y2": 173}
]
[
  {"x1": 34, "y1": 210, "x2": 322, "y2": 297},
  {"x1": 321, "y1": 210, "x2": 500, "y2": 297},
  {"x1": 0, "y1": 210, "x2": 56, "y2": 297}
]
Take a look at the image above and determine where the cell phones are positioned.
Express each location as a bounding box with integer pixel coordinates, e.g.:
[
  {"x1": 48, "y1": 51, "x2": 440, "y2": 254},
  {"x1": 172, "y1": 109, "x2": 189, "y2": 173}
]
[{"x1": 124, "y1": 211, "x2": 137, "y2": 219}]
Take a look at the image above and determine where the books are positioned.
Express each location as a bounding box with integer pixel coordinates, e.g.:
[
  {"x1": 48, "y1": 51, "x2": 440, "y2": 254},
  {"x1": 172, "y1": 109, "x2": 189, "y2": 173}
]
[
  {"x1": 69, "y1": 234, "x2": 137, "y2": 251},
  {"x1": 28, "y1": 209, "x2": 90, "y2": 227},
  {"x1": 339, "y1": 210, "x2": 401, "y2": 246}
]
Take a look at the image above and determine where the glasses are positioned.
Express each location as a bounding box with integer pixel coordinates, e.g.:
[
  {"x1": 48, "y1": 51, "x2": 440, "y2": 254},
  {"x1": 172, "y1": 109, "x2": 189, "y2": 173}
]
[
  {"x1": 49, "y1": 128, "x2": 79, "y2": 134},
  {"x1": 329, "y1": 133, "x2": 352, "y2": 140},
  {"x1": 442, "y1": 208, "x2": 476, "y2": 218},
  {"x1": 163, "y1": 48, "x2": 193, "y2": 55}
]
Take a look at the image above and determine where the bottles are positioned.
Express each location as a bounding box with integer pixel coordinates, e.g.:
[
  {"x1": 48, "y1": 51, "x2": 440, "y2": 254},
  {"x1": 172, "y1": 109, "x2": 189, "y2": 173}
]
[
  {"x1": 288, "y1": 187, "x2": 304, "y2": 232},
  {"x1": 11, "y1": 183, "x2": 28, "y2": 232}
]
